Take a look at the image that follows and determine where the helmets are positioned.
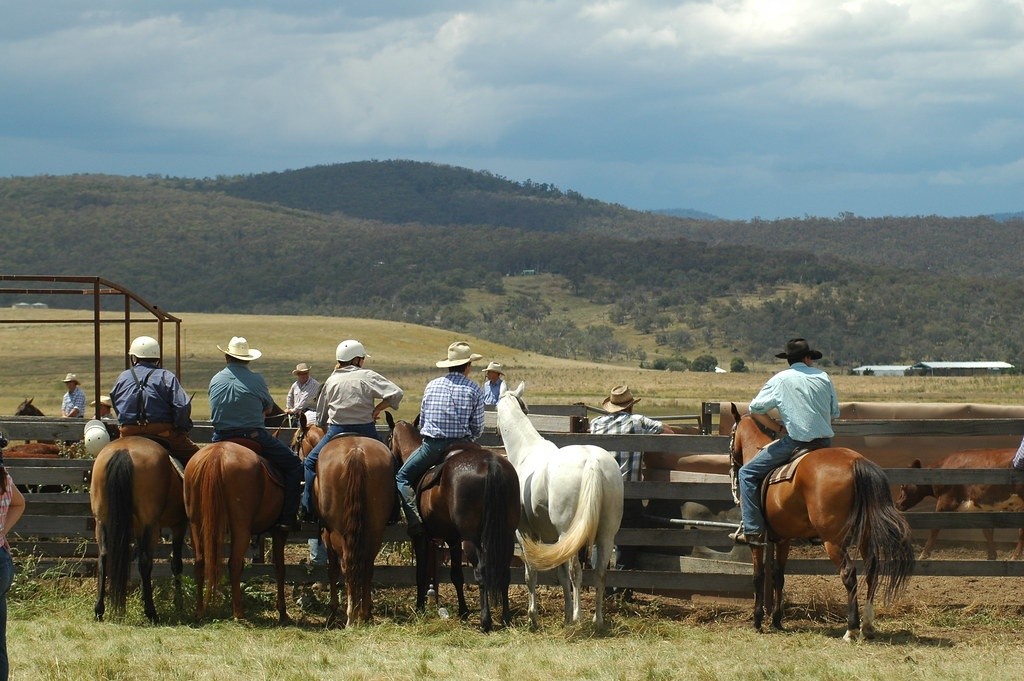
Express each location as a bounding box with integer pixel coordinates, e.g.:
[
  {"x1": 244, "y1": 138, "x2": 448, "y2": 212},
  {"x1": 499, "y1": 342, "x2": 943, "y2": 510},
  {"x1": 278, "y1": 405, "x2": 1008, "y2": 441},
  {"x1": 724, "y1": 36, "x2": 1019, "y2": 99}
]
[
  {"x1": 128, "y1": 336, "x2": 160, "y2": 359},
  {"x1": 335, "y1": 339, "x2": 365, "y2": 361}
]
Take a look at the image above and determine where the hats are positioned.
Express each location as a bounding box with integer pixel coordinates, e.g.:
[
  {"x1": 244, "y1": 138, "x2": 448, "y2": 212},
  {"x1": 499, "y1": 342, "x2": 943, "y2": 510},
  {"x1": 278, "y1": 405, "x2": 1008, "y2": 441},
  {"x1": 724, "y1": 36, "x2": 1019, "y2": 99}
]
[
  {"x1": 775, "y1": 338, "x2": 822, "y2": 360},
  {"x1": 602, "y1": 385, "x2": 640, "y2": 412},
  {"x1": 90, "y1": 396, "x2": 112, "y2": 407},
  {"x1": 62, "y1": 373, "x2": 80, "y2": 385},
  {"x1": 217, "y1": 336, "x2": 262, "y2": 360},
  {"x1": 292, "y1": 363, "x2": 312, "y2": 375},
  {"x1": 482, "y1": 362, "x2": 504, "y2": 376},
  {"x1": 436, "y1": 341, "x2": 483, "y2": 367}
]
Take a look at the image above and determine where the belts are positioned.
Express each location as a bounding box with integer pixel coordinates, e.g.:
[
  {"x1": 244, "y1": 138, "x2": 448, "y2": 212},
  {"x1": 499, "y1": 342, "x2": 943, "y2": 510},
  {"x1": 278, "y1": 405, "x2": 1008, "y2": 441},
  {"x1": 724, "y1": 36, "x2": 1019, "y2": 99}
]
[{"x1": 216, "y1": 427, "x2": 255, "y2": 434}]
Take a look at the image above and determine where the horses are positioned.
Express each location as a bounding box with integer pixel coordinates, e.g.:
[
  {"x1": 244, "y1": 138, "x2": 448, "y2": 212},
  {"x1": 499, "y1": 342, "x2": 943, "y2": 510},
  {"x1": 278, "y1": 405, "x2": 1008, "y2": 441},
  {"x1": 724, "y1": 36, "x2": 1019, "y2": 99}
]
[
  {"x1": 385, "y1": 410, "x2": 521, "y2": 636},
  {"x1": 2, "y1": 397, "x2": 61, "y2": 457},
  {"x1": 496, "y1": 378, "x2": 625, "y2": 631},
  {"x1": 89, "y1": 435, "x2": 187, "y2": 624},
  {"x1": 182, "y1": 439, "x2": 293, "y2": 629},
  {"x1": 729, "y1": 403, "x2": 913, "y2": 643},
  {"x1": 292, "y1": 411, "x2": 397, "y2": 630}
]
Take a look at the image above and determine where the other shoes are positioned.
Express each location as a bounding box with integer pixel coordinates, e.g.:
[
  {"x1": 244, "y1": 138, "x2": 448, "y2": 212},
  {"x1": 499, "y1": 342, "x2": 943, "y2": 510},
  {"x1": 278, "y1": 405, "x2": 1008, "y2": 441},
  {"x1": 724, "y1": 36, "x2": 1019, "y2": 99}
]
[{"x1": 729, "y1": 533, "x2": 762, "y2": 549}]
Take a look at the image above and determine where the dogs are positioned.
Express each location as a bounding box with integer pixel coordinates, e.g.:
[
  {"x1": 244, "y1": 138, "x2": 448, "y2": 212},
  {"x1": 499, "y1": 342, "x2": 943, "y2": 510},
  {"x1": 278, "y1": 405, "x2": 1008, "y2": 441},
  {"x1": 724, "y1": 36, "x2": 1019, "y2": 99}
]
[{"x1": 896, "y1": 448, "x2": 1024, "y2": 560}]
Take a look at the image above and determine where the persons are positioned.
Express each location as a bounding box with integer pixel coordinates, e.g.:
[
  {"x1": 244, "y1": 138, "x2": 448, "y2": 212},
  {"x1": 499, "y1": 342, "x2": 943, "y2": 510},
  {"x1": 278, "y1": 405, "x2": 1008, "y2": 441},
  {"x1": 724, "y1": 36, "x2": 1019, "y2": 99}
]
[
  {"x1": 89, "y1": 396, "x2": 121, "y2": 443},
  {"x1": 728, "y1": 338, "x2": 840, "y2": 545},
  {"x1": 1012, "y1": 436, "x2": 1024, "y2": 471},
  {"x1": 482, "y1": 362, "x2": 508, "y2": 405},
  {"x1": 395, "y1": 341, "x2": 485, "y2": 536},
  {"x1": 590, "y1": 386, "x2": 674, "y2": 601},
  {"x1": 61, "y1": 372, "x2": 86, "y2": 445},
  {"x1": 284, "y1": 363, "x2": 321, "y2": 427},
  {"x1": 0, "y1": 431, "x2": 25, "y2": 681},
  {"x1": 208, "y1": 336, "x2": 302, "y2": 533},
  {"x1": 298, "y1": 340, "x2": 403, "y2": 523},
  {"x1": 110, "y1": 335, "x2": 199, "y2": 470}
]
